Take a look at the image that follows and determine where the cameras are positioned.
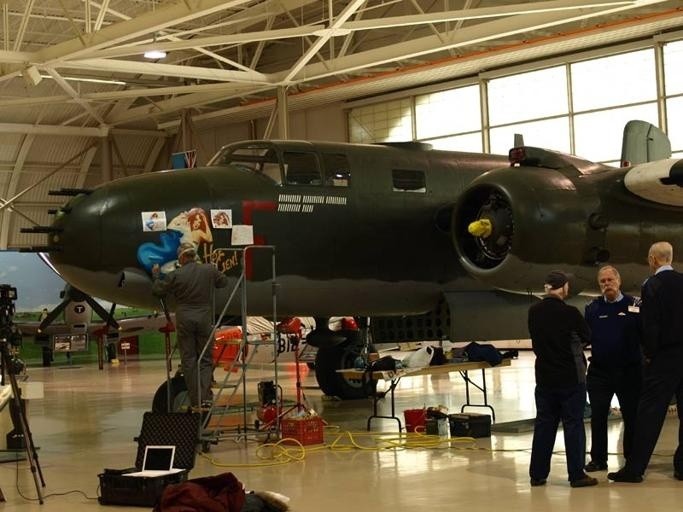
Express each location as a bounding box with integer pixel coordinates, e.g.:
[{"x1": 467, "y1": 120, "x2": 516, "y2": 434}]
[{"x1": 0, "y1": 284, "x2": 23, "y2": 346}]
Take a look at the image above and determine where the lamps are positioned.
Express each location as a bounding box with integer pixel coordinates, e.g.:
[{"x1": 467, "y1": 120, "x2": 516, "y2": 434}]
[
  {"x1": 20, "y1": 64, "x2": 43, "y2": 86},
  {"x1": 144, "y1": 33, "x2": 167, "y2": 59}
]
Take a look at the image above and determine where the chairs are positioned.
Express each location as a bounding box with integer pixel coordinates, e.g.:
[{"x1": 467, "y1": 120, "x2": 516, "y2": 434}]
[
  {"x1": 332, "y1": 170, "x2": 349, "y2": 186},
  {"x1": 286, "y1": 155, "x2": 313, "y2": 184}
]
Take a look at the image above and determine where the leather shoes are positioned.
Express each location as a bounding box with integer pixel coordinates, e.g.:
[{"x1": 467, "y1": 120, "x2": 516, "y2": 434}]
[
  {"x1": 674, "y1": 470, "x2": 683, "y2": 481},
  {"x1": 607, "y1": 468, "x2": 643, "y2": 483},
  {"x1": 584, "y1": 459, "x2": 607, "y2": 472}
]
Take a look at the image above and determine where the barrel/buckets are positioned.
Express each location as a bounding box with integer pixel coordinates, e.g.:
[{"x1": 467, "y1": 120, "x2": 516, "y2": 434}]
[{"x1": 404, "y1": 408, "x2": 427, "y2": 432}]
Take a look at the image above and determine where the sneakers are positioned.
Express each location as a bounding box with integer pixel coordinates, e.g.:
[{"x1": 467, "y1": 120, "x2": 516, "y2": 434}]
[
  {"x1": 530, "y1": 478, "x2": 546, "y2": 486},
  {"x1": 570, "y1": 477, "x2": 598, "y2": 488}
]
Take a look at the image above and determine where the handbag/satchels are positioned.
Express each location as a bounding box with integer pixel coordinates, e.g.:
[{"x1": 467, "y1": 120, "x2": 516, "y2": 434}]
[{"x1": 366, "y1": 356, "x2": 396, "y2": 370}]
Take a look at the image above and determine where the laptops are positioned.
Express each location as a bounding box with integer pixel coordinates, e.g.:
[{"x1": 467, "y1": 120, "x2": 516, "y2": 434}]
[{"x1": 121, "y1": 445, "x2": 176, "y2": 478}]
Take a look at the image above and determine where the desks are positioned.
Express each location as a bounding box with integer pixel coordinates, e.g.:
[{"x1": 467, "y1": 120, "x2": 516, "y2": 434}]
[{"x1": 335, "y1": 355, "x2": 511, "y2": 433}]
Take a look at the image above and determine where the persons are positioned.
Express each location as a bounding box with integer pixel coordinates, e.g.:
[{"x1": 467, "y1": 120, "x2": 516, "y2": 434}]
[
  {"x1": 152, "y1": 244, "x2": 227, "y2": 412},
  {"x1": 584, "y1": 265, "x2": 642, "y2": 472},
  {"x1": 527, "y1": 272, "x2": 597, "y2": 488},
  {"x1": 607, "y1": 240, "x2": 683, "y2": 483},
  {"x1": 150, "y1": 213, "x2": 159, "y2": 230},
  {"x1": 214, "y1": 212, "x2": 230, "y2": 227},
  {"x1": 160, "y1": 207, "x2": 214, "y2": 264}
]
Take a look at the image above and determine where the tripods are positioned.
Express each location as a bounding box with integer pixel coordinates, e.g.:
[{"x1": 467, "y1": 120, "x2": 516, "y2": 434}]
[{"x1": 0, "y1": 339, "x2": 46, "y2": 505}]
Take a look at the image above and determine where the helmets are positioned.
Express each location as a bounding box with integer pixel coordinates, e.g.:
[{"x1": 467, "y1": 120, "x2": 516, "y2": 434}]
[{"x1": 177, "y1": 242, "x2": 195, "y2": 258}]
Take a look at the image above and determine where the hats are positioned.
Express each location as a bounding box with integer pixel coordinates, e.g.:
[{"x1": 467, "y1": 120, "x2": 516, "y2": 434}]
[{"x1": 543, "y1": 271, "x2": 568, "y2": 290}]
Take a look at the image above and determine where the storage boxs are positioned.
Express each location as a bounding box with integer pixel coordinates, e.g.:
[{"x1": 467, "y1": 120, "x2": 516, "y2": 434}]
[
  {"x1": 98, "y1": 412, "x2": 199, "y2": 508},
  {"x1": 448, "y1": 412, "x2": 491, "y2": 438}
]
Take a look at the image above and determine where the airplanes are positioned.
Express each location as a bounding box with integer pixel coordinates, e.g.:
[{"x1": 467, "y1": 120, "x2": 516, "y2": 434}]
[
  {"x1": 17, "y1": 120, "x2": 682, "y2": 398},
  {"x1": 13, "y1": 269, "x2": 177, "y2": 371}
]
